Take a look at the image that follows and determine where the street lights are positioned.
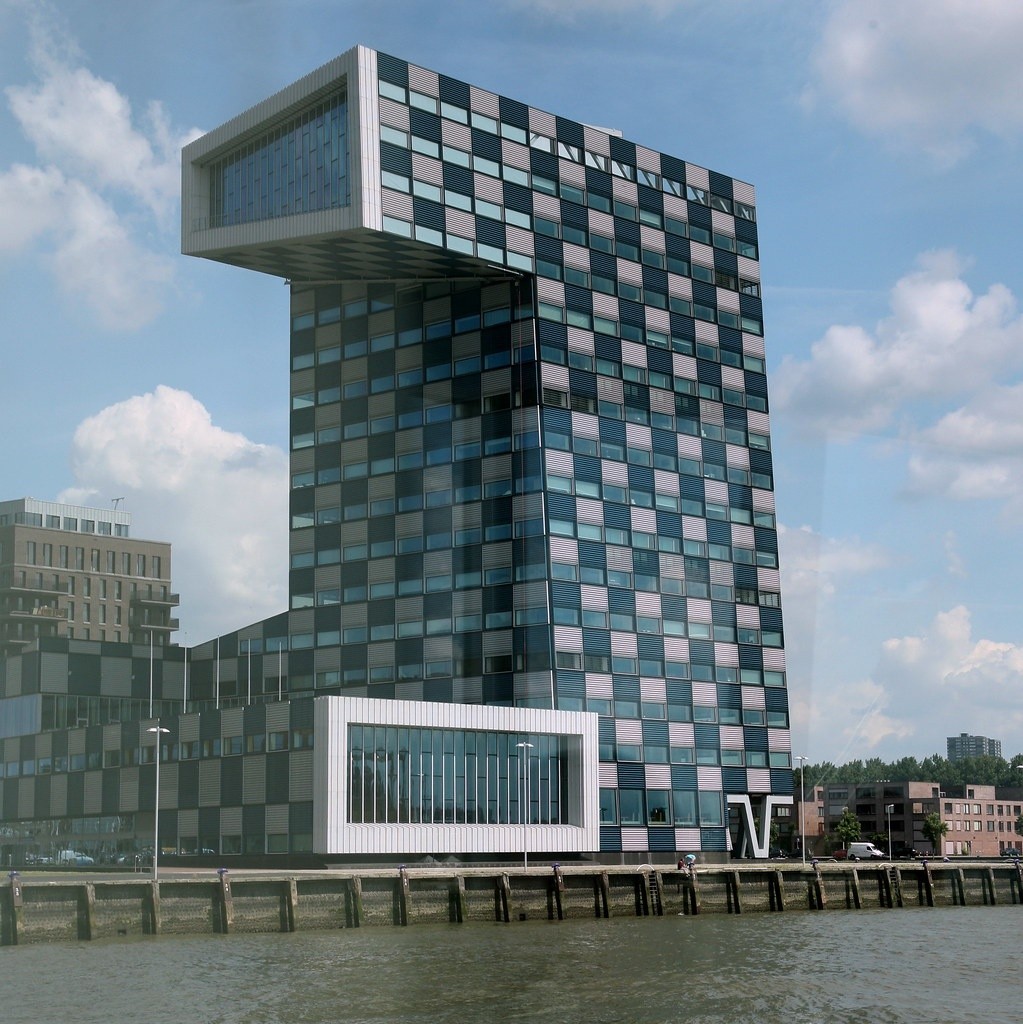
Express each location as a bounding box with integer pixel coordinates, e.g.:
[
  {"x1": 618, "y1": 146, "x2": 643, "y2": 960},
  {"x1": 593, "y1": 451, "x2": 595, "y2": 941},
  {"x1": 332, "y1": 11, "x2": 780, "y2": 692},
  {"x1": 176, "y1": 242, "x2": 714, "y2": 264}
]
[
  {"x1": 514, "y1": 741, "x2": 535, "y2": 872},
  {"x1": 145, "y1": 726, "x2": 170, "y2": 880},
  {"x1": 793, "y1": 755, "x2": 809, "y2": 867},
  {"x1": 887, "y1": 804, "x2": 894, "y2": 861}
]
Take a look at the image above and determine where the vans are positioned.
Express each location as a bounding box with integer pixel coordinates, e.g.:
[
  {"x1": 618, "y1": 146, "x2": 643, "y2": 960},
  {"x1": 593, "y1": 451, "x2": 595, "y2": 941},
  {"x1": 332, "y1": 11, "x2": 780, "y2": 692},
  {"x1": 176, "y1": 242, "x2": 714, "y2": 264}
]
[{"x1": 846, "y1": 842, "x2": 885, "y2": 860}]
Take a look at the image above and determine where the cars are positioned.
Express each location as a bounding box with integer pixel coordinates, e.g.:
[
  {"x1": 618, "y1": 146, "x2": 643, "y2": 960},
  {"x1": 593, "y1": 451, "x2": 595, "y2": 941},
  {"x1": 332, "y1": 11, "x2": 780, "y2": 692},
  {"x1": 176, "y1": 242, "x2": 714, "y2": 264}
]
[
  {"x1": 25, "y1": 844, "x2": 154, "y2": 868},
  {"x1": 832, "y1": 849, "x2": 847, "y2": 861},
  {"x1": 1001, "y1": 847, "x2": 1021, "y2": 856}
]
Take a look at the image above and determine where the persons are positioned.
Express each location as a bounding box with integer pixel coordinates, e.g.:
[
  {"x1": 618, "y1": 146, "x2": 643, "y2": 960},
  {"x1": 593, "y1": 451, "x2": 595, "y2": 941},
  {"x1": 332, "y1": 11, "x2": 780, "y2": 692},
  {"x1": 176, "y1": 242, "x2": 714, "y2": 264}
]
[{"x1": 678, "y1": 858, "x2": 690, "y2": 878}]
